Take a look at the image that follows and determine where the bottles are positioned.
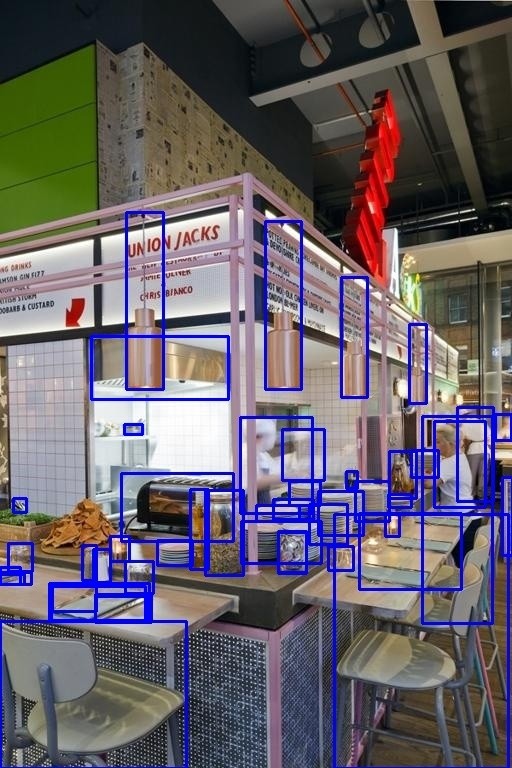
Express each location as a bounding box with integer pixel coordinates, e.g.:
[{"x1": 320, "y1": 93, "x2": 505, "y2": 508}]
[
  {"x1": 94, "y1": 549, "x2": 111, "y2": 583},
  {"x1": 191, "y1": 489, "x2": 208, "y2": 570},
  {"x1": 205, "y1": 490, "x2": 244, "y2": 575},
  {"x1": 346, "y1": 472, "x2": 357, "y2": 489},
  {"x1": 83, "y1": 546, "x2": 97, "y2": 580},
  {"x1": 390, "y1": 451, "x2": 417, "y2": 498}
]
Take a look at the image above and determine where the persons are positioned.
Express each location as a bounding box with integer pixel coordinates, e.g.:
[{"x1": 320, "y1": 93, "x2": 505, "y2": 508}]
[
  {"x1": 457, "y1": 408, "x2": 494, "y2": 501},
  {"x1": 422, "y1": 416, "x2": 493, "y2": 623},
  {"x1": 282, "y1": 430, "x2": 324, "y2": 480},
  {"x1": 240, "y1": 417, "x2": 312, "y2": 513},
  {"x1": 495, "y1": 415, "x2": 511, "y2": 441}
]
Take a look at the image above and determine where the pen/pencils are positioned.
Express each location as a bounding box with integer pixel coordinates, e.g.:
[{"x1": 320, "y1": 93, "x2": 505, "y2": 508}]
[
  {"x1": 0, "y1": 575, "x2": 24, "y2": 584},
  {"x1": 96, "y1": 596, "x2": 148, "y2": 623}
]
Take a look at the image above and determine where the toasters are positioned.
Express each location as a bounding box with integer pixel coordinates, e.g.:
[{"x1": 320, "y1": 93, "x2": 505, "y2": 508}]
[{"x1": 121, "y1": 474, "x2": 233, "y2": 542}]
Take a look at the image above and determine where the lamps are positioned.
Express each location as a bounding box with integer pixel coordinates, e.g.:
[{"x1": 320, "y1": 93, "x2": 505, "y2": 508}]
[
  {"x1": 127, "y1": 212, "x2": 163, "y2": 389},
  {"x1": 266, "y1": 222, "x2": 302, "y2": 390},
  {"x1": 342, "y1": 278, "x2": 368, "y2": 398},
  {"x1": 409, "y1": 324, "x2": 426, "y2": 404}
]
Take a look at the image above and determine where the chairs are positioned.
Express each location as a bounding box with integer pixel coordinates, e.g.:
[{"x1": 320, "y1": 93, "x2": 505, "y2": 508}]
[
  {"x1": 0, "y1": 622, "x2": 186, "y2": 768},
  {"x1": 335, "y1": 514, "x2": 509, "y2": 768}
]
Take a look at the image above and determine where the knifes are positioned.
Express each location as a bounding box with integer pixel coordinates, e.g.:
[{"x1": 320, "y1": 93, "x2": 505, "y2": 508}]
[
  {"x1": 1, "y1": 572, "x2": 31, "y2": 585},
  {"x1": 96, "y1": 594, "x2": 152, "y2": 622}
]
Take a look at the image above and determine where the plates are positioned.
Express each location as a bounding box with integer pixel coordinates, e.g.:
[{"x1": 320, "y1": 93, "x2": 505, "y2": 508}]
[
  {"x1": 257, "y1": 506, "x2": 299, "y2": 521},
  {"x1": 158, "y1": 541, "x2": 192, "y2": 566},
  {"x1": 242, "y1": 521, "x2": 321, "y2": 563},
  {"x1": 411, "y1": 451, "x2": 439, "y2": 478},
  {"x1": 356, "y1": 482, "x2": 390, "y2": 521},
  {"x1": 318, "y1": 492, "x2": 364, "y2": 535},
  {"x1": 273, "y1": 499, "x2": 313, "y2": 520},
  {"x1": 388, "y1": 494, "x2": 412, "y2": 507},
  {"x1": 290, "y1": 482, "x2": 320, "y2": 505},
  {"x1": 309, "y1": 504, "x2": 347, "y2": 545}
]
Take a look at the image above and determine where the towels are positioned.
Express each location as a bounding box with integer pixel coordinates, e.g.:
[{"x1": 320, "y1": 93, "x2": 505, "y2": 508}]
[
  {"x1": 0, "y1": 567, "x2": 21, "y2": 585},
  {"x1": 51, "y1": 586, "x2": 145, "y2": 620}
]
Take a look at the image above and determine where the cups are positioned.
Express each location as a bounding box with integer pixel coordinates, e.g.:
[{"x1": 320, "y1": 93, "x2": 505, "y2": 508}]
[
  {"x1": 126, "y1": 561, "x2": 154, "y2": 594},
  {"x1": 329, "y1": 546, "x2": 354, "y2": 571},
  {"x1": 385, "y1": 515, "x2": 399, "y2": 536},
  {"x1": 278, "y1": 533, "x2": 306, "y2": 573},
  {"x1": 110, "y1": 536, "x2": 129, "y2": 561},
  {"x1": 8, "y1": 544, "x2": 33, "y2": 571}
]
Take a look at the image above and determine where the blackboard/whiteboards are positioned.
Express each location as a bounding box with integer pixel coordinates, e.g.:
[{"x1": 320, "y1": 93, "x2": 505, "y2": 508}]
[{"x1": 503, "y1": 478, "x2": 512, "y2": 556}]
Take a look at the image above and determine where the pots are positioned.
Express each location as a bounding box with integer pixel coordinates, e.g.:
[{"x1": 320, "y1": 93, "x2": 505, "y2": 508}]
[{"x1": 124, "y1": 425, "x2": 143, "y2": 434}]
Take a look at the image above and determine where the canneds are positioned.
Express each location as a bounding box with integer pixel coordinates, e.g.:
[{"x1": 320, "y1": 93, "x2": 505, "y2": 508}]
[{"x1": 279, "y1": 531, "x2": 307, "y2": 573}]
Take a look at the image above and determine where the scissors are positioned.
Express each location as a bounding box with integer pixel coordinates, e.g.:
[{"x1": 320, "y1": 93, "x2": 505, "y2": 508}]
[{"x1": 13, "y1": 498, "x2": 27, "y2": 512}]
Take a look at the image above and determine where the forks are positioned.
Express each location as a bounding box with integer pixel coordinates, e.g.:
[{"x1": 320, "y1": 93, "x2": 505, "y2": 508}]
[
  {"x1": 53, "y1": 583, "x2": 97, "y2": 611},
  {"x1": 359, "y1": 514, "x2": 461, "y2": 589}
]
